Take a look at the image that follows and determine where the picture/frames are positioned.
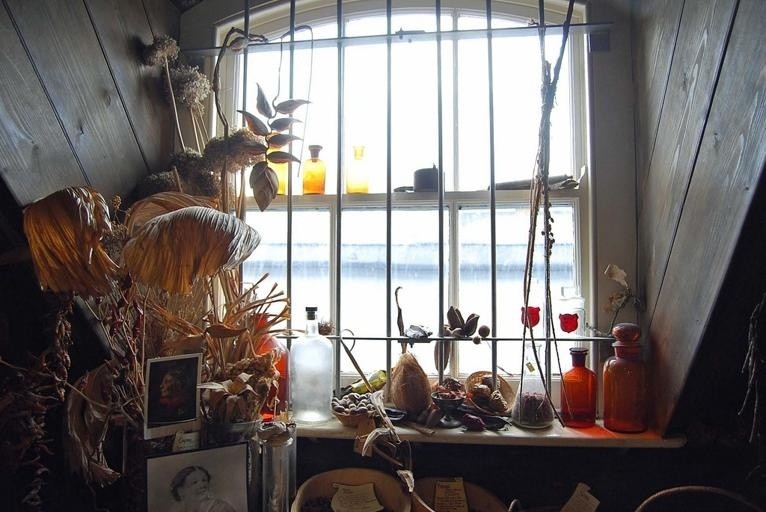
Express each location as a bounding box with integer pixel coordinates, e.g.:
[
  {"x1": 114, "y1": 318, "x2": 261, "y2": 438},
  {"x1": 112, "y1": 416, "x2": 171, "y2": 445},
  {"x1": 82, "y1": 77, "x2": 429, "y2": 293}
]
[
  {"x1": 141, "y1": 352, "x2": 203, "y2": 440},
  {"x1": 141, "y1": 441, "x2": 252, "y2": 512}
]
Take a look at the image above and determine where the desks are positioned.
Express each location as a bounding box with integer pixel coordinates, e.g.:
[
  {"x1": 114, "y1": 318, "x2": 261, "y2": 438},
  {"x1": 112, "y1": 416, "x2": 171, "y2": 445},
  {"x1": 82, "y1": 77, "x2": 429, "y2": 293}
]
[{"x1": 292, "y1": 410, "x2": 687, "y2": 512}]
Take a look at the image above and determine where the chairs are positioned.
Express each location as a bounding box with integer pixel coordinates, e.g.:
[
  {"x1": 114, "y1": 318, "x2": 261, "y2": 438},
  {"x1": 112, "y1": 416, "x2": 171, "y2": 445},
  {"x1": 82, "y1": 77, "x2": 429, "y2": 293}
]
[
  {"x1": 412, "y1": 477, "x2": 509, "y2": 511},
  {"x1": 290, "y1": 467, "x2": 412, "y2": 512}
]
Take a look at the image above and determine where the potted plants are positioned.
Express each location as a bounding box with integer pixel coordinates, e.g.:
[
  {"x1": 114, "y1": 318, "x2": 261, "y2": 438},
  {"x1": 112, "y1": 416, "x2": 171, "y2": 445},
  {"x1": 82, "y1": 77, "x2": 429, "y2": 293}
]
[
  {"x1": 206, "y1": 418, "x2": 264, "y2": 439},
  {"x1": 200, "y1": 346, "x2": 283, "y2": 439}
]
